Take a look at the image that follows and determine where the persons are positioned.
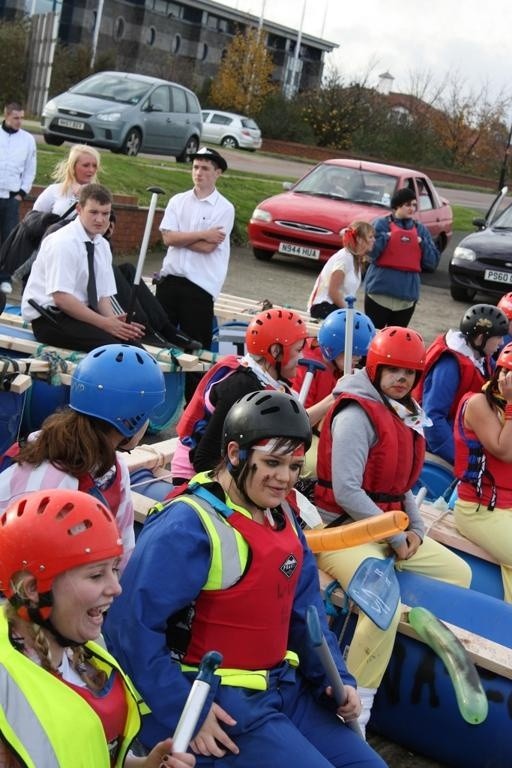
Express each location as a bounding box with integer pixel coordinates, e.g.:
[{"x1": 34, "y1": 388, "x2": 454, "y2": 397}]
[
  {"x1": 416, "y1": 179, "x2": 424, "y2": 195},
  {"x1": 1, "y1": 102, "x2": 37, "y2": 250},
  {"x1": 306, "y1": 220, "x2": 376, "y2": 319},
  {"x1": 103, "y1": 210, "x2": 203, "y2": 352},
  {"x1": 363, "y1": 188, "x2": 441, "y2": 330},
  {"x1": 20, "y1": 142, "x2": 116, "y2": 298},
  {"x1": 326, "y1": 170, "x2": 352, "y2": 197},
  {"x1": 369, "y1": 183, "x2": 395, "y2": 202},
  {"x1": 155, "y1": 147, "x2": 235, "y2": 351},
  {"x1": 21, "y1": 183, "x2": 147, "y2": 353}
]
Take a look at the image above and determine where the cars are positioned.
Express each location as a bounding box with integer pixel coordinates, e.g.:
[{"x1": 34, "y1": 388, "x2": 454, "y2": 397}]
[
  {"x1": 245, "y1": 154, "x2": 457, "y2": 278},
  {"x1": 37, "y1": 68, "x2": 205, "y2": 165},
  {"x1": 445, "y1": 185, "x2": 512, "y2": 308},
  {"x1": 197, "y1": 108, "x2": 264, "y2": 154}
]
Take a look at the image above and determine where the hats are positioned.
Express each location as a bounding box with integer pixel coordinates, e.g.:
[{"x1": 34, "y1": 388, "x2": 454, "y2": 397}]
[
  {"x1": 184, "y1": 146, "x2": 229, "y2": 173},
  {"x1": 388, "y1": 187, "x2": 418, "y2": 209}
]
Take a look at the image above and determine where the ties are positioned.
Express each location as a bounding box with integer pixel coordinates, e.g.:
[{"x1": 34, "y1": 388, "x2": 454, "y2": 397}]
[{"x1": 84, "y1": 240, "x2": 101, "y2": 311}]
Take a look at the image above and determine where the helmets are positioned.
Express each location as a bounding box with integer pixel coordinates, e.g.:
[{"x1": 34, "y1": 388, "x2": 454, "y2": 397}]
[
  {"x1": 244, "y1": 308, "x2": 308, "y2": 369},
  {"x1": 316, "y1": 308, "x2": 376, "y2": 364},
  {"x1": 458, "y1": 292, "x2": 512, "y2": 372},
  {"x1": 220, "y1": 389, "x2": 313, "y2": 458},
  {"x1": 0, "y1": 487, "x2": 124, "y2": 633},
  {"x1": 365, "y1": 325, "x2": 427, "y2": 391},
  {"x1": 68, "y1": 342, "x2": 166, "y2": 445}
]
